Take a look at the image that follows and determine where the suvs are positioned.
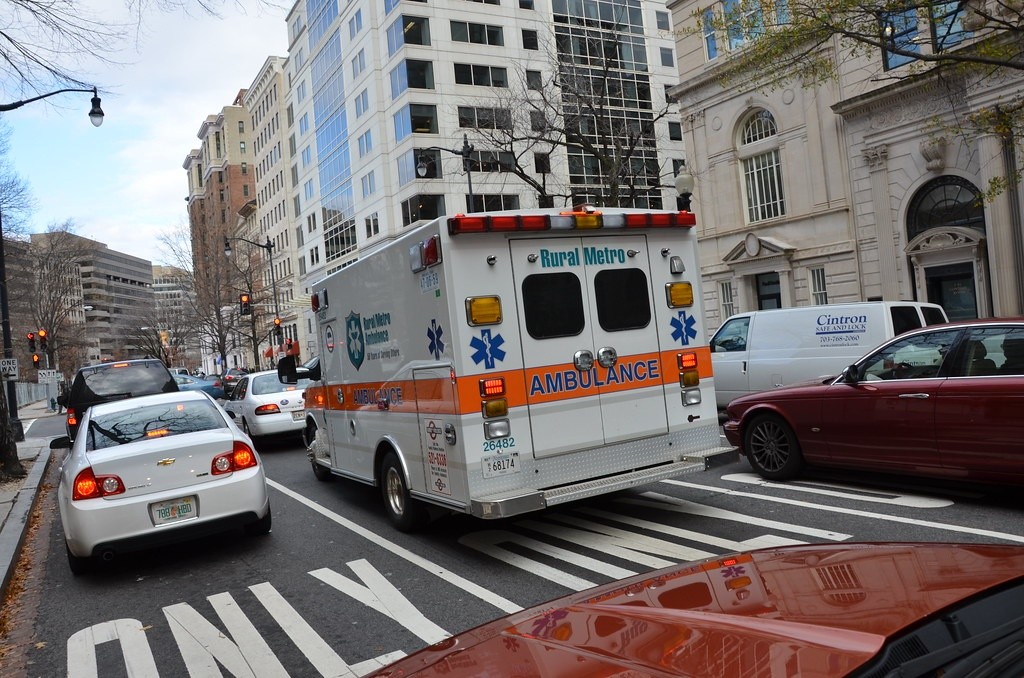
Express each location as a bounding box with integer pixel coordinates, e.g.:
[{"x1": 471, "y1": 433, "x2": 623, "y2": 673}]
[{"x1": 57, "y1": 355, "x2": 180, "y2": 440}]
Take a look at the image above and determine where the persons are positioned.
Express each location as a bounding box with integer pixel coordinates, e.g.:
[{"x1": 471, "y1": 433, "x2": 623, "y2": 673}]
[{"x1": 892, "y1": 353, "x2": 939, "y2": 378}]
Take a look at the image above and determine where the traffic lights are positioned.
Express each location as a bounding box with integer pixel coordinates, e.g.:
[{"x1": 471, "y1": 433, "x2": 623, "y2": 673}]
[
  {"x1": 33, "y1": 354, "x2": 40, "y2": 370},
  {"x1": 239, "y1": 294, "x2": 251, "y2": 315},
  {"x1": 274, "y1": 318, "x2": 281, "y2": 336},
  {"x1": 38, "y1": 329, "x2": 48, "y2": 351},
  {"x1": 28, "y1": 333, "x2": 36, "y2": 352}
]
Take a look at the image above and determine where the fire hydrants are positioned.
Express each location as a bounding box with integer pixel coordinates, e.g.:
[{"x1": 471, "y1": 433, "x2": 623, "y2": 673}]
[{"x1": 50, "y1": 396, "x2": 57, "y2": 412}]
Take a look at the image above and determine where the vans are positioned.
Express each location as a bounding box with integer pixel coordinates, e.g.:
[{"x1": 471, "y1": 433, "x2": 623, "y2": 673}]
[{"x1": 709, "y1": 301, "x2": 950, "y2": 410}]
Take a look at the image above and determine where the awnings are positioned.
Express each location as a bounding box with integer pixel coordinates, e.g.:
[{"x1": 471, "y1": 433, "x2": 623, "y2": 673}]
[{"x1": 264, "y1": 341, "x2": 299, "y2": 357}]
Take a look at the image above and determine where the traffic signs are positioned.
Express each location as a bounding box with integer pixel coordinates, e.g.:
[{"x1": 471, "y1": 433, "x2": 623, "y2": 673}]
[{"x1": 0, "y1": 359, "x2": 19, "y2": 381}]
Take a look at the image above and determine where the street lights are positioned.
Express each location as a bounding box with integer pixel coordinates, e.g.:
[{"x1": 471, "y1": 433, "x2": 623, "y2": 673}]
[
  {"x1": 224, "y1": 236, "x2": 282, "y2": 352},
  {"x1": 673, "y1": 166, "x2": 695, "y2": 211},
  {"x1": 0, "y1": 87, "x2": 104, "y2": 443},
  {"x1": 53, "y1": 305, "x2": 94, "y2": 371},
  {"x1": 417, "y1": 133, "x2": 474, "y2": 213},
  {"x1": 141, "y1": 326, "x2": 176, "y2": 367}
]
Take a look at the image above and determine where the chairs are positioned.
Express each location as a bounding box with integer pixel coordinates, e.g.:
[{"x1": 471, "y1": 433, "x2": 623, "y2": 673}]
[{"x1": 969, "y1": 341, "x2": 997, "y2": 375}]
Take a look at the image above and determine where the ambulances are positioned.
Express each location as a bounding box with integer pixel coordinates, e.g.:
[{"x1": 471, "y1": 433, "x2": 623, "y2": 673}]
[{"x1": 278, "y1": 204, "x2": 722, "y2": 532}]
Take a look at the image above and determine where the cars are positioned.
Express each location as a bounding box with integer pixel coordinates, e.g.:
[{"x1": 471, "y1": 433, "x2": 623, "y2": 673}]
[
  {"x1": 49, "y1": 389, "x2": 272, "y2": 573},
  {"x1": 362, "y1": 543, "x2": 1024, "y2": 677},
  {"x1": 723, "y1": 315, "x2": 1024, "y2": 490},
  {"x1": 168, "y1": 356, "x2": 321, "y2": 443}
]
